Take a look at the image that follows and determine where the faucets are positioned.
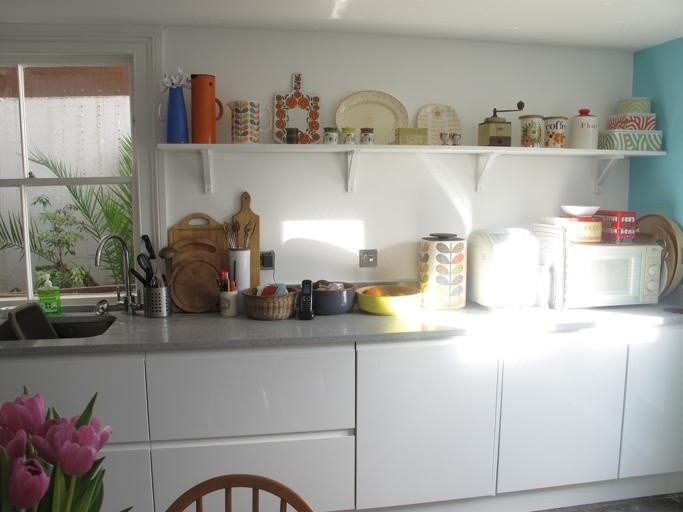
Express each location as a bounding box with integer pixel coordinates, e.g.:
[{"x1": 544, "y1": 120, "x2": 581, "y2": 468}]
[{"x1": 93, "y1": 233, "x2": 132, "y2": 314}]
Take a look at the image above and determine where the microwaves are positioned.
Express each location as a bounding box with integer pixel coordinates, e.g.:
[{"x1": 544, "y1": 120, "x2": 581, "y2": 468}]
[{"x1": 563, "y1": 241, "x2": 663, "y2": 310}]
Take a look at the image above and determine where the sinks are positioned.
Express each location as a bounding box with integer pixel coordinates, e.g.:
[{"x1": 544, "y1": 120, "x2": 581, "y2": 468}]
[{"x1": 0, "y1": 313, "x2": 118, "y2": 341}]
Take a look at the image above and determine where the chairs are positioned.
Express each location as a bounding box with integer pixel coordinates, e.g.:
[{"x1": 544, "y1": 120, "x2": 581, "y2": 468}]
[{"x1": 161, "y1": 472, "x2": 312, "y2": 512}]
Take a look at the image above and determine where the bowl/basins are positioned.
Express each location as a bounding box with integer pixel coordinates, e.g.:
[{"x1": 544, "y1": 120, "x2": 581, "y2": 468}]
[
  {"x1": 311, "y1": 282, "x2": 356, "y2": 315},
  {"x1": 355, "y1": 285, "x2": 422, "y2": 316},
  {"x1": 561, "y1": 204, "x2": 601, "y2": 219}
]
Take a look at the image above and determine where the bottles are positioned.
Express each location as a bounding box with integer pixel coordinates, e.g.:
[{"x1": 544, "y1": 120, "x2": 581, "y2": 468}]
[
  {"x1": 342, "y1": 128, "x2": 357, "y2": 144},
  {"x1": 322, "y1": 127, "x2": 338, "y2": 144},
  {"x1": 519, "y1": 115, "x2": 568, "y2": 148},
  {"x1": 571, "y1": 108, "x2": 599, "y2": 150},
  {"x1": 360, "y1": 127, "x2": 374, "y2": 144}
]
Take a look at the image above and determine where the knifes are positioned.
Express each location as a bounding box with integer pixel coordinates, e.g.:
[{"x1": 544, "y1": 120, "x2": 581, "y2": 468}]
[{"x1": 142, "y1": 234, "x2": 165, "y2": 287}]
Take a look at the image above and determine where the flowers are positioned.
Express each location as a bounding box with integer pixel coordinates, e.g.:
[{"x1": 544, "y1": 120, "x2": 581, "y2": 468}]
[{"x1": 0, "y1": 389, "x2": 110, "y2": 508}]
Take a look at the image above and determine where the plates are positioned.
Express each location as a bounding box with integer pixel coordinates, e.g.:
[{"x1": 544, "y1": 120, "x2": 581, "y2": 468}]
[
  {"x1": 335, "y1": 91, "x2": 410, "y2": 145},
  {"x1": 417, "y1": 103, "x2": 462, "y2": 145}
]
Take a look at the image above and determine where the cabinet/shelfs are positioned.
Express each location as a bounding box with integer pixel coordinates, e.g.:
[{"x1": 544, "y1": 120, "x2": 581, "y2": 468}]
[
  {"x1": 621, "y1": 330, "x2": 683, "y2": 502},
  {"x1": 355, "y1": 338, "x2": 496, "y2": 507},
  {"x1": 146, "y1": 341, "x2": 357, "y2": 511},
  {"x1": 498, "y1": 335, "x2": 628, "y2": 498},
  {"x1": 3, "y1": 350, "x2": 155, "y2": 512}
]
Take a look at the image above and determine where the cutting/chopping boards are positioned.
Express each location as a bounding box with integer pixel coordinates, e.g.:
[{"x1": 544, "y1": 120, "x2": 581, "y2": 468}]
[
  {"x1": 167, "y1": 213, "x2": 227, "y2": 313},
  {"x1": 233, "y1": 192, "x2": 260, "y2": 288}
]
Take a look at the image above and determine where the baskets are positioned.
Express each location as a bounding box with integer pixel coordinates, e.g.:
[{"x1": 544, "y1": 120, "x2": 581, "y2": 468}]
[{"x1": 242, "y1": 283, "x2": 299, "y2": 320}]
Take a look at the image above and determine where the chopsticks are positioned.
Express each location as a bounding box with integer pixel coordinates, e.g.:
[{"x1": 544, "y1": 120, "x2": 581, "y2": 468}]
[{"x1": 227, "y1": 220, "x2": 256, "y2": 249}]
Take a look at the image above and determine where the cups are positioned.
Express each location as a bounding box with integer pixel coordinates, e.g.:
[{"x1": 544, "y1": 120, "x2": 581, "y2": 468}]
[
  {"x1": 190, "y1": 74, "x2": 224, "y2": 144},
  {"x1": 441, "y1": 132, "x2": 461, "y2": 146},
  {"x1": 286, "y1": 128, "x2": 303, "y2": 143},
  {"x1": 227, "y1": 100, "x2": 272, "y2": 143}
]
